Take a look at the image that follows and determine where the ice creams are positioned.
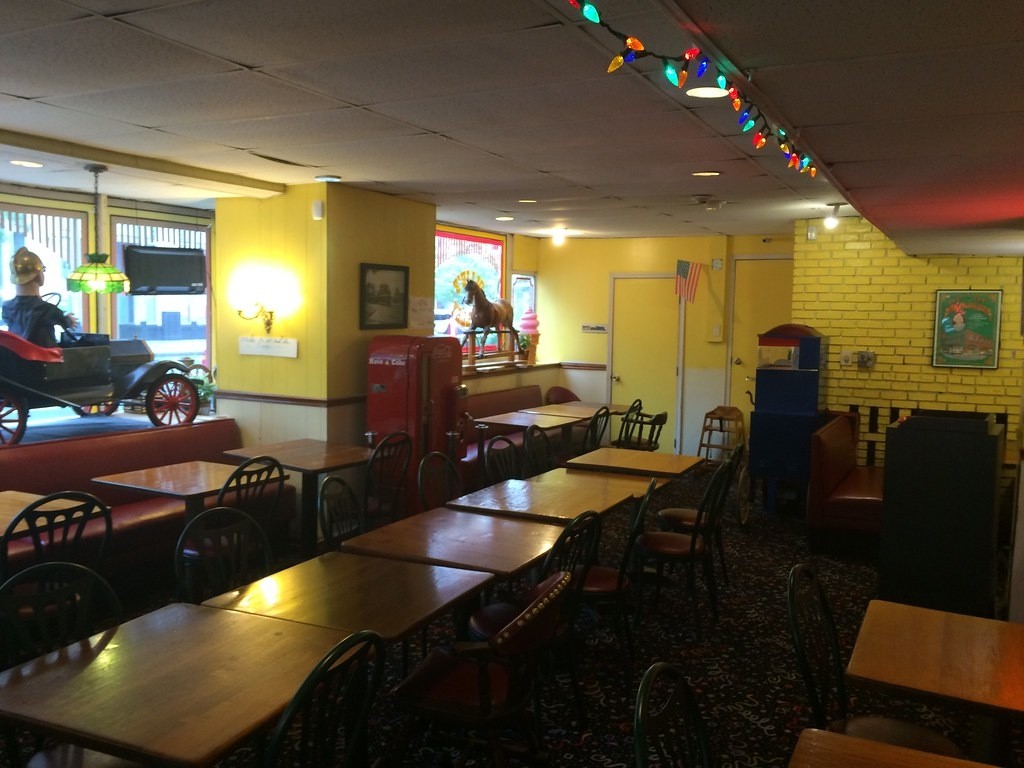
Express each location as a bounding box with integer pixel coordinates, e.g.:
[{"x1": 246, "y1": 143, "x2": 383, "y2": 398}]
[{"x1": 518, "y1": 306, "x2": 540, "y2": 365}]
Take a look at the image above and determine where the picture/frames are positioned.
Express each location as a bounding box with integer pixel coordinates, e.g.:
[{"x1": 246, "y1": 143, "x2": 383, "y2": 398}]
[{"x1": 932, "y1": 289, "x2": 1003, "y2": 370}]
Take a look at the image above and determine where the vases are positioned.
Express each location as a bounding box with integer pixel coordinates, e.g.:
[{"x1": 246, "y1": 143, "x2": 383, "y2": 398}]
[{"x1": 360, "y1": 262, "x2": 410, "y2": 329}]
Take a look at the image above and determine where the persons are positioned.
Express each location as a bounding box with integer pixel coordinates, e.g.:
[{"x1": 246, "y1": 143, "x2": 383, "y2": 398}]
[{"x1": 3, "y1": 248, "x2": 79, "y2": 345}]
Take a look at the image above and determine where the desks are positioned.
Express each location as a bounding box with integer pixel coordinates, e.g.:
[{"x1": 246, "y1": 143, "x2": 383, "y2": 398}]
[
  {"x1": 447, "y1": 478, "x2": 634, "y2": 616},
  {"x1": 0, "y1": 603, "x2": 388, "y2": 768},
  {"x1": 0, "y1": 490, "x2": 113, "y2": 602},
  {"x1": 788, "y1": 728, "x2": 1002, "y2": 768},
  {"x1": 517, "y1": 400, "x2": 643, "y2": 440},
  {"x1": 566, "y1": 447, "x2": 704, "y2": 478},
  {"x1": 341, "y1": 507, "x2": 588, "y2": 581},
  {"x1": 525, "y1": 466, "x2": 675, "y2": 584},
  {"x1": 224, "y1": 439, "x2": 397, "y2": 560},
  {"x1": 473, "y1": 412, "x2": 583, "y2": 476},
  {"x1": 91, "y1": 460, "x2": 291, "y2": 605},
  {"x1": 201, "y1": 551, "x2": 497, "y2": 768},
  {"x1": 846, "y1": 600, "x2": 1024, "y2": 713}
]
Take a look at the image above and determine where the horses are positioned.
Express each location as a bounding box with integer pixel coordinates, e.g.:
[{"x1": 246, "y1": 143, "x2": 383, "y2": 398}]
[{"x1": 461, "y1": 278, "x2": 525, "y2": 359}]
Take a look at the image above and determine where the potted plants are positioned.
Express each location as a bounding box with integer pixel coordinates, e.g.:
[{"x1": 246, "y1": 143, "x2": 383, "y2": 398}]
[
  {"x1": 519, "y1": 333, "x2": 532, "y2": 360},
  {"x1": 185, "y1": 384, "x2": 216, "y2": 415}
]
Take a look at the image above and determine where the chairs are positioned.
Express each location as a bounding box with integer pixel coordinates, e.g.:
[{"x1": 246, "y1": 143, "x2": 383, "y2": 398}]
[
  {"x1": 697, "y1": 406, "x2": 746, "y2": 472},
  {"x1": 0, "y1": 399, "x2": 641, "y2": 768},
  {"x1": 786, "y1": 564, "x2": 963, "y2": 758},
  {"x1": 265, "y1": 443, "x2": 745, "y2": 768},
  {"x1": 611, "y1": 412, "x2": 667, "y2": 452},
  {"x1": 634, "y1": 661, "x2": 713, "y2": 768},
  {"x1": 546, "y1": 387, "x2": 579, "y2": 405}
]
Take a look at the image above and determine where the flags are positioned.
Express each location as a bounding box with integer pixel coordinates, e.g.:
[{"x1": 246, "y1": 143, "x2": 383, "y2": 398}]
[{"x1": 674, "y1": 259, "x2": 701, "y2": 303}]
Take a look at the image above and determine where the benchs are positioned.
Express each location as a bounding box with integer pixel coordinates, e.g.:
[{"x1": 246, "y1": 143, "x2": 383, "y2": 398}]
[
  {"x1": 0, "y1": 331, "x2": 65, "y2": 383},
  {"x1": 460, "y1": 386, "x2": 589, "y2": 493},
  {"x1": 806, "y1": 415, "x2": 885, "y2": 556},
  {"x1": 0, "y1": 417, "x2": 295, "y2": 602}
]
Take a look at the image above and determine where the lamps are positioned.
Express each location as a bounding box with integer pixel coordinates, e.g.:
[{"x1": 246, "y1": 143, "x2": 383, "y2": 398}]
[{"x1": 66, "y1": 163, "x2": 131, "y2": 295}]
